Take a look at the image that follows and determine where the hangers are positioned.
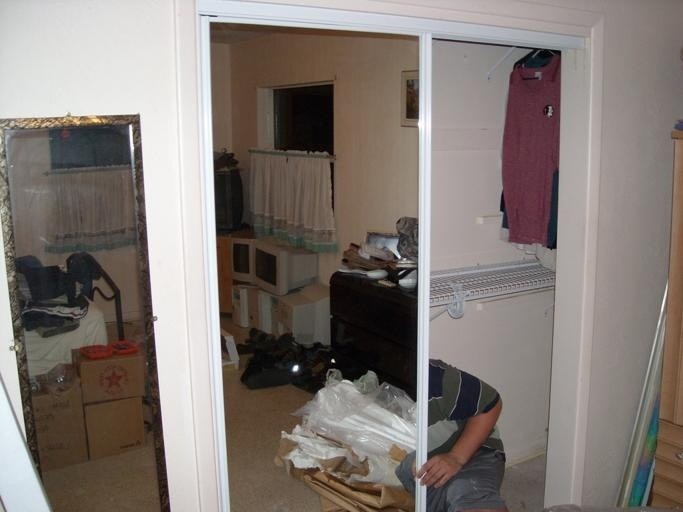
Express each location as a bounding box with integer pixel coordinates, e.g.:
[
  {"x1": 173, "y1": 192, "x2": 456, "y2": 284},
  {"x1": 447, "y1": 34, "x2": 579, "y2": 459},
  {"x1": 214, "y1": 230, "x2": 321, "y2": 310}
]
[{"x1": 515, "y1": 48, "x2": 558, "y2": 69}]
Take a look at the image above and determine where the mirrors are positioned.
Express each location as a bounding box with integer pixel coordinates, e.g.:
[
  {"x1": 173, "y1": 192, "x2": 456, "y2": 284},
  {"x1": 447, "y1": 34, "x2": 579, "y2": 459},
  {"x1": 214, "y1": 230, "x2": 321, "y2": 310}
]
[
  {"x1": 1, "y1": 114, "x2": 173, "y2": 511},
  {"x1": 203, "y1": 16, "x2": 433, "y2": 512}
]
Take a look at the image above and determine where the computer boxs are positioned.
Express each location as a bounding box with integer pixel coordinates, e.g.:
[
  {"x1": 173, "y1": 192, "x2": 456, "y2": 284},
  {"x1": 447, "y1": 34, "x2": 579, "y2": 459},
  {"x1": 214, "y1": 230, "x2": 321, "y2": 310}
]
[
  {"x1": 229, "y1": 287, "x2": 248, "y2": 328},
  {"x1": 256, "y1": 290, "x2": 271, "y2": 333}
]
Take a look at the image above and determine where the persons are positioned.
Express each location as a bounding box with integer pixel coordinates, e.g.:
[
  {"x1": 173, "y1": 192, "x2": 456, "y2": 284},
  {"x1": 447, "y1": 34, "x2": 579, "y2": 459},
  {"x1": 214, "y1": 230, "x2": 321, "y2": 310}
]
[
  {"x1": 395, "y1": 450, "x2": 416, "y2": 495},
  {"x1": 417, "y1": 359, "x2": 508, "y2": 512}
]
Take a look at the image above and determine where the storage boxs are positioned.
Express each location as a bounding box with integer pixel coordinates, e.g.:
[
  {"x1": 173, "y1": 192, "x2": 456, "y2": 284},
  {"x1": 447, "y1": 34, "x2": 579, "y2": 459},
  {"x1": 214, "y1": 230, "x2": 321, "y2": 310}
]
[{"x1": 31, "y1": 343, "x2": 147, "y2": 473}]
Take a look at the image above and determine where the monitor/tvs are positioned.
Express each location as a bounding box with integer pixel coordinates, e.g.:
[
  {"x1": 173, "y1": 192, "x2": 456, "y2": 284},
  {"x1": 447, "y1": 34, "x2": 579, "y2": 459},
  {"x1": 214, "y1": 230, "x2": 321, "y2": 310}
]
[
  {"x1": 255, "y1": 243, "x2": 319, "y2": 296},
  {"x1": 228, "y1": 236, "x2": 270, "y2": 285},
  {"x1": 278, "y1": 281, "x2": 329, "y2": 348}
]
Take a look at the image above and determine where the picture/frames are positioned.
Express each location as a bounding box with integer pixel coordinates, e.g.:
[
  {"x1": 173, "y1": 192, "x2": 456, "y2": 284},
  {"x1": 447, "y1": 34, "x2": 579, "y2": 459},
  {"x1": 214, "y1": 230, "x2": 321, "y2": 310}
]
[{"x1": 399, "y1": 70, "x2": 419, "y2": 128}]
